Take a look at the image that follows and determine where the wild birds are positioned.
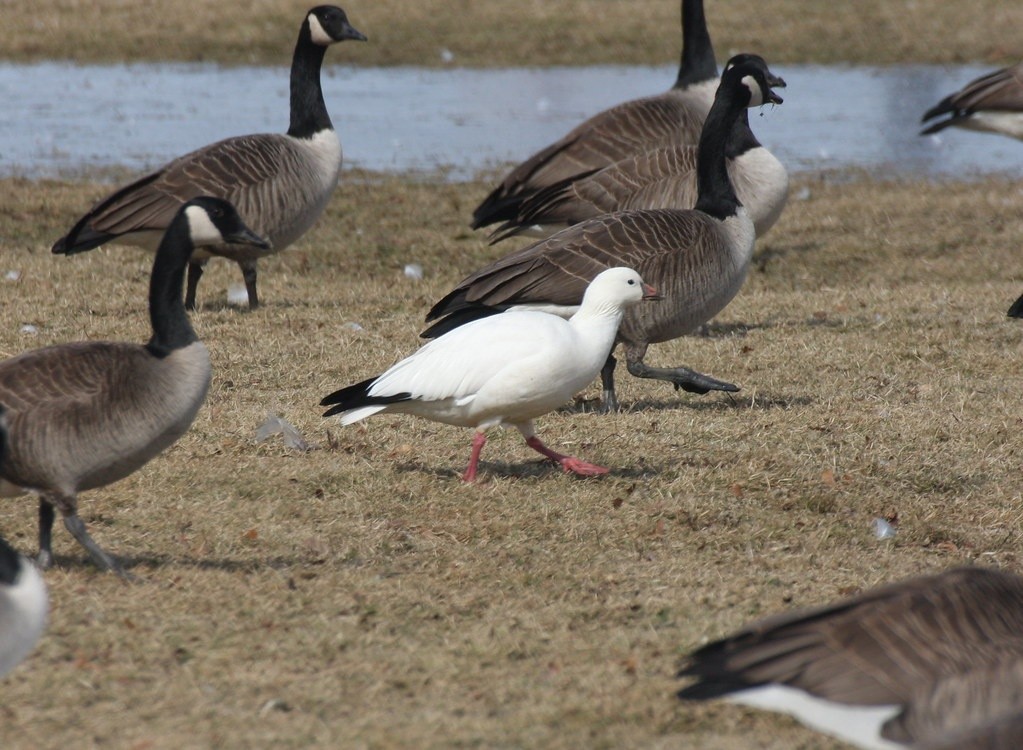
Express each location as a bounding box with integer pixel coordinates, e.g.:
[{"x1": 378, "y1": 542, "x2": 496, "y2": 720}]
[
  {"x1": 319, "y1": 267, "x2": 665, "y2": 483},
  {"x1": 673, "y1": 564, "x2": 1023, "y2": 750},
  {"x1": 0, "y1": 196, "x2": 270, "y2": 581},
  {"x1": 50, "y1": 6, "x2": 369, "y2": 310},
  {"x1": 920, "y1": 62, "x2": 1023, "y2": 143},
  {"x1": 421, "y1": 68, "x2": 792, "y2": 411},
  {"x1": 0, "y1": 539, "x2": 53, "y2": 681},
  {"x1": 485, "y1": 53, "x2": 788, "y2": 247},
  {"x1": 466, "y1": 0, "x2": 722, "y2": 234}
]
